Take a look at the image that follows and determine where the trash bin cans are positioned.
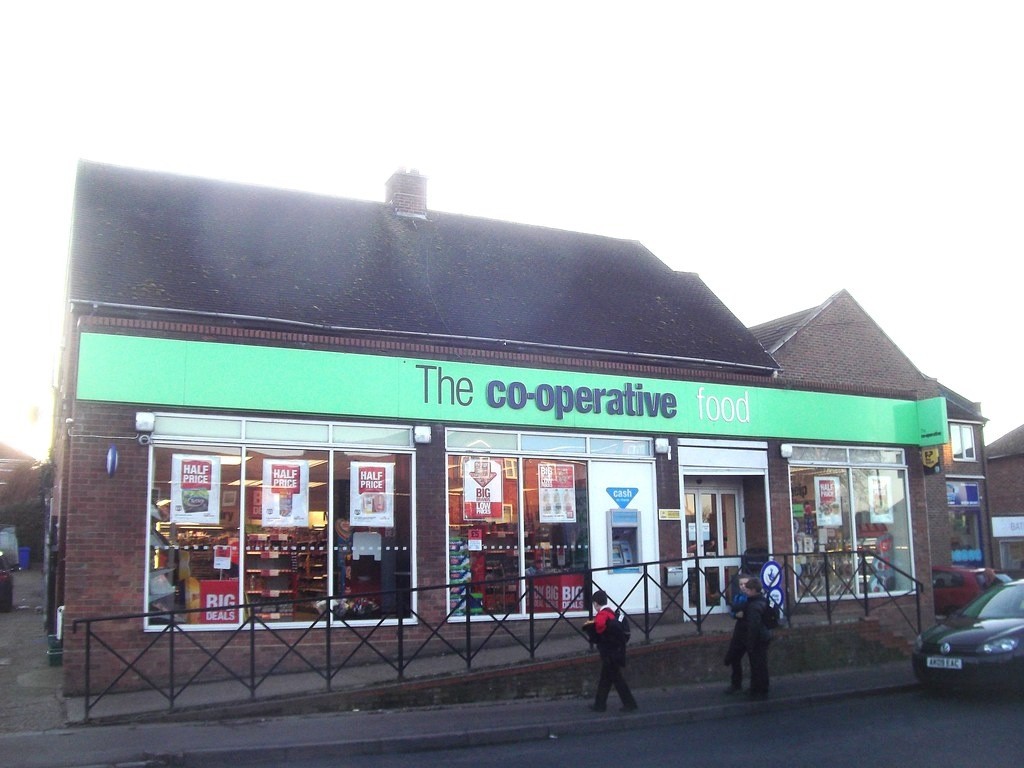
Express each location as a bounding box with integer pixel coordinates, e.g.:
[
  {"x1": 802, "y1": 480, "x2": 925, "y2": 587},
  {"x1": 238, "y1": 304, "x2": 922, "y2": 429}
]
[{"x1": 18, "y1": 547, "x2": 31, "y2": 569}]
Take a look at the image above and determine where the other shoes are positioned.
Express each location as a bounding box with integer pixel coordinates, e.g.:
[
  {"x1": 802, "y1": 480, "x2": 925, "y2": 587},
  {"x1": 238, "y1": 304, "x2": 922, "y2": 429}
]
[
  {"x1": 746, "y1": 687, "x2": 767, "y2": 700},
  {"x1": 619, "y1": 704, "x2": 637, "y2": 712},
  {"x1": 588, "y1": 703, "x2": 604, "y2": 711},
  {"x1": 726, "y1": 682, "x2": 740, "y2": 695}
]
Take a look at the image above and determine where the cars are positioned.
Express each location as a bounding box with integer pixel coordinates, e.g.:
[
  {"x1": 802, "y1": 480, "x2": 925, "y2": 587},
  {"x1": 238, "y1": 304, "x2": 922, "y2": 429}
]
[
  {"x1": 0, "y1": 551, "x2": 23, "y2": 611},
  {"x1": 911, "y1": 568, "x2": 1024, "y2": 694},
  {"x1": 844, "y1": 536, "x2": 877, "y2": 555}
]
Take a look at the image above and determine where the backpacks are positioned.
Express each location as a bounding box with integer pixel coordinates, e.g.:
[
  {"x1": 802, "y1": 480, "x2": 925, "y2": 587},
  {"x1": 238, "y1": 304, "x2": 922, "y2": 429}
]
[{"x1": 757, "y1": 601, "x2": 780, "y2": 629}]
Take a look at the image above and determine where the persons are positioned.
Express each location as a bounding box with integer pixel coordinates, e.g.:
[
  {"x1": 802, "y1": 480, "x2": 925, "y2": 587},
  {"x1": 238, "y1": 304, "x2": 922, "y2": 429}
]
[
  {"x1": 982, "y1": 569, "x2": 1003, "y2": 590},
  {"x1": 723, "y1": 573, "x2": 772, "y2": 699},
  {"x1": 581, "y1": 590, "x2": 638, "y2": 713},
  {"x1": 526, "y1": 559, "x2": 544, "y2": 575}
]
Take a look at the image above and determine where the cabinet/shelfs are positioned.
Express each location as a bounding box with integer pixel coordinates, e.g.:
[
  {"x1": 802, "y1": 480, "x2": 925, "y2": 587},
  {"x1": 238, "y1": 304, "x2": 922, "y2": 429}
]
[
  {"x1": 162, "y1": 534, "x2": 343, "y2": 620},
  {"x1": 483, "y1": 546, "x2": 541, "y2": 608}
]
